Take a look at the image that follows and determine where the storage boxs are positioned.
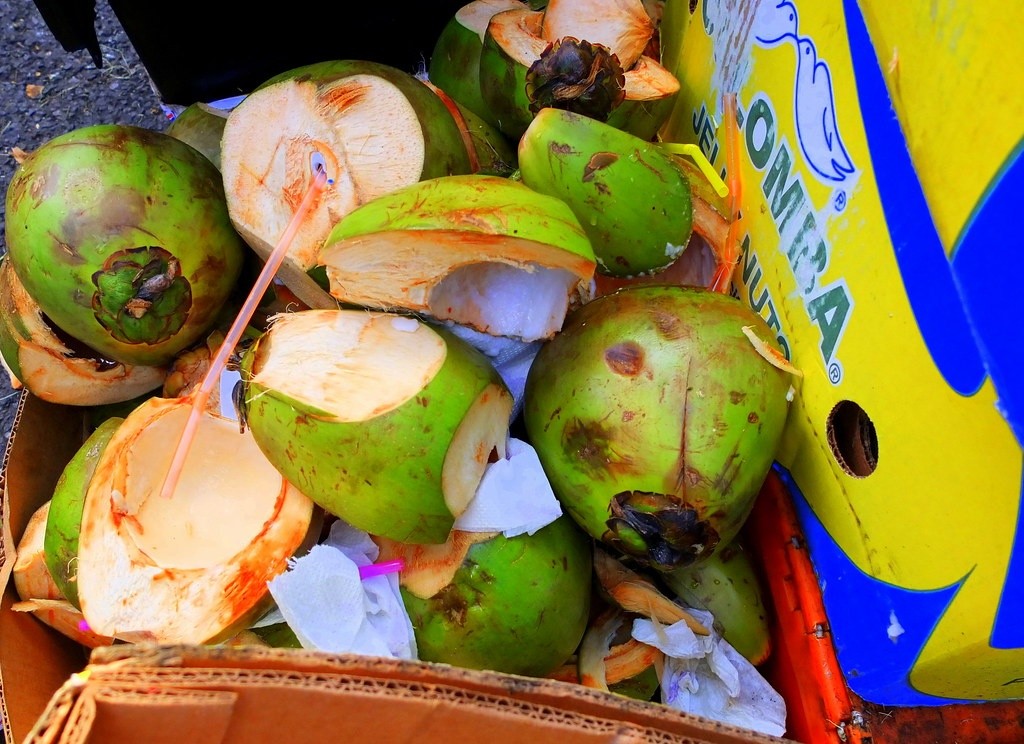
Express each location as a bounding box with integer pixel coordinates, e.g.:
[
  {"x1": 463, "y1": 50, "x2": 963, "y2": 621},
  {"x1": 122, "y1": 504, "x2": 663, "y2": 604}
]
[
  {"x1": 858, "y1": 0, "x2": 1024, "y2": 447},
  {"x1": 23, "y1": 644, "x2": 798, "y2": 744},
  {"x1": 753, "y1": 469, "x2": 1024, "y2": 744},
  {"x1": 662, "y1": 0, "x2": 1024, "y2": 711}
]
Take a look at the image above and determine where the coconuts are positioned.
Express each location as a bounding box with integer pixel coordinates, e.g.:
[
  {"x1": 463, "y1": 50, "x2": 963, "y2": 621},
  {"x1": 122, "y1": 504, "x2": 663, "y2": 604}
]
[{"x1": 4, "y1": 0, "x2": 789, "y2": 705}]
